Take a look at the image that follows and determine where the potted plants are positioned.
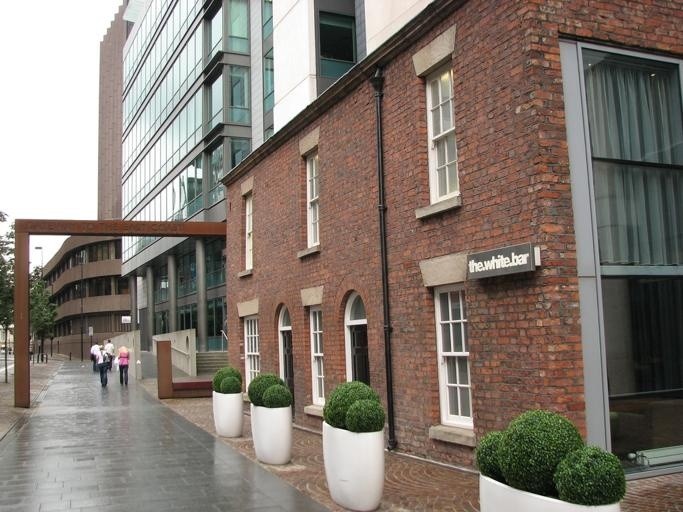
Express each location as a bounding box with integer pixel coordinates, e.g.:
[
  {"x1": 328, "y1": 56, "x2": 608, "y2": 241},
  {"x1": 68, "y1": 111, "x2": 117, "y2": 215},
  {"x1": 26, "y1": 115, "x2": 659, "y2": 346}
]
[
  {"x1": 212, "y1": 367, "x2": 385, "y2": 510},
  {"x1": 477, "y1": 409, "x2": 625, "y2": 512}
]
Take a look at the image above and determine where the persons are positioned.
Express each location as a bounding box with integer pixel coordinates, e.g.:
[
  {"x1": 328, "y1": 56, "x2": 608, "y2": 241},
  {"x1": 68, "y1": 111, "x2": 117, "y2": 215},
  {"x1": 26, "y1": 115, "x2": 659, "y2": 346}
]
[
  {"x1": 118, "y1": 345, "x2": 130, "y2": 387},
  {"x1": 90, "y1": 341, "x2": 101, "y2": 372},
  {"x1": 103, "y1": 339, "x2": 114, "y2": 371},
  {"x1": 96, "y1": 344, "x2": 111, "y2": 388}
]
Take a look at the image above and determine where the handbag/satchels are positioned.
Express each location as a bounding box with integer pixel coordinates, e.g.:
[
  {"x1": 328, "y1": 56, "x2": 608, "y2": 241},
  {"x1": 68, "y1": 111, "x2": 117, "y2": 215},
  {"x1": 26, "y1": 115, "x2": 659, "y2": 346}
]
[{"x1": 103, "y1": 352, "x2": 112, "y2": 368}]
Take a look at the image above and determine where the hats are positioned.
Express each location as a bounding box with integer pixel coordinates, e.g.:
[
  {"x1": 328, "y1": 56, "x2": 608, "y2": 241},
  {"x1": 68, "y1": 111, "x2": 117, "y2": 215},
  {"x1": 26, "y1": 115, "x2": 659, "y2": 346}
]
[{"x1": 118, "y1": 346, "x2": 129, "y2": 352}]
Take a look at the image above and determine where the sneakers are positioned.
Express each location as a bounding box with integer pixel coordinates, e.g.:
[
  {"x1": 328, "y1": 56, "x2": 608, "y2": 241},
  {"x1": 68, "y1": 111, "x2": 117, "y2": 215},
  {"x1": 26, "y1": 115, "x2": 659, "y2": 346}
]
[
  {"x1": 102, "y1": 384, "x2": 107, "y2": 387},
  {"x1": 120, "y1": 383, "x2": 128, "y2": 385}
]
